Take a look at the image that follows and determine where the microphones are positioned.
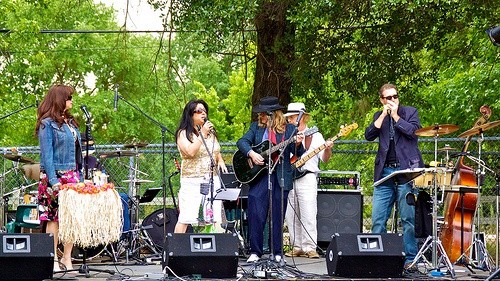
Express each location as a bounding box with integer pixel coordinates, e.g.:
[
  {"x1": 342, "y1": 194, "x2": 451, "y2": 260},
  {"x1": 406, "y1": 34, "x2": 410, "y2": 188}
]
[
  {"x1": 296, "y1": 108, "x2": 306, "y2": 124},
  {"x1": 81, "y1": 105, "x2": 91, "y2": 118},
  {"x1": 450, "y1": 151, "x2": 474, "y2": 157},
  {"x1": 204, "y1": 117, "x2": 215, "y2": 133},
  {"x1": 269, "y1": 112, "x2": 273, "y2": 121}
]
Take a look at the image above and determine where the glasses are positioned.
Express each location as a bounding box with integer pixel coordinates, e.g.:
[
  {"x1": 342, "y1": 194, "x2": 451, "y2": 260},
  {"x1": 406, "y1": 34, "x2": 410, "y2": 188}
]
[
  {"x1": 193, "y1": 107, "x2": 207, "y2": 114},
  {"x1": 381, "y1": 94, "x2": 400, "y2": 100},
  {"x1": 67, "y1": 95, "x2": 72, "y2": 100}
]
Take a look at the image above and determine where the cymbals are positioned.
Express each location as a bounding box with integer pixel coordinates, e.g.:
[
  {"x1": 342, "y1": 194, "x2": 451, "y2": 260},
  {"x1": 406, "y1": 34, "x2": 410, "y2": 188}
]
[
  {"x1": 97, "y1": 150, "x2": 138, "y2": 159},
  {"x1": 437, "y1": 147, "x2": 456, "y2": 150},
  {"x1": 124, "y1": 143, "x2": 148, "y2": 148},
  {"x1": 457, "y1": 119, "x2": 500, "y2": 139},
  {"x1": 415, "y1": 124, "x2": 461, "y2": 137},
  {"x1": 3, "y1": 153, "x2": 37, "y2": 164}
]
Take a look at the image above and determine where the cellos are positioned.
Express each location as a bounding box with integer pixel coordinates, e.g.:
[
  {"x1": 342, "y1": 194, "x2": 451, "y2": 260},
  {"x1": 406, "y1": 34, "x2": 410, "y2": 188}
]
[{"x1": 436, "y1": 105, "x2": 492, "y2": 266}]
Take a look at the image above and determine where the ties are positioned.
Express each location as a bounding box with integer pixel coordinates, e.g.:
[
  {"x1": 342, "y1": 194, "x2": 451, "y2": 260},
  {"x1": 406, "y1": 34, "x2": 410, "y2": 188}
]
[{"x1": 268, "y1": 131, "x2": 278, "y2": 163}]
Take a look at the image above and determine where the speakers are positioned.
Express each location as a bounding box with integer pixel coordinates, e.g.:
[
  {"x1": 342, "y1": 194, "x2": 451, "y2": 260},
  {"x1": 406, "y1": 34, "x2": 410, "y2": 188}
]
[
  {"x1": 0, "y1": 233, "x2": 53, "y2": 281},
  {"x1": 162, "y1": 232, "x2": 239, "y2": 278},
  {"x1": 317, "y1": 191, "x2": 406, "y2": 278}
]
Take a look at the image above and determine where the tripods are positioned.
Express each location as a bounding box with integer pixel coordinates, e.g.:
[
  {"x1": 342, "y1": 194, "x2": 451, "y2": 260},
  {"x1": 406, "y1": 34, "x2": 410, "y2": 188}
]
[
  {"x1": 238, "y1": 119, "x2": 305, "y2": 278},
  {"x1": 406, "y1": 128, "x2": 495, "y2": 278},
  {"x1": 118, "y1": 146, "x2": 161, "y2": 262},
  {"x1": 53, "y1": 118, "x2": 115, "y2": 278}
]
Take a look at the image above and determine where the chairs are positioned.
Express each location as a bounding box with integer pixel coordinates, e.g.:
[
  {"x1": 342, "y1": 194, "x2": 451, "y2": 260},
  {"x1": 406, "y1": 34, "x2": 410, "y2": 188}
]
[{"x1": 6, "y1": 204, "x2": 41, "y2": 233}]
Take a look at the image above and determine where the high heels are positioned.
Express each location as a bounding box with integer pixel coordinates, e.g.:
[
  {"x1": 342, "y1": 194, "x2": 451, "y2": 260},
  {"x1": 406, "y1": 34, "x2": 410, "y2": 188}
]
[
  {"x1": 53, "y1": 258, "x2": 62, "y2": 272},
  {"x1": 60, "y1": 259, "x2": 78, "y2": 273}
]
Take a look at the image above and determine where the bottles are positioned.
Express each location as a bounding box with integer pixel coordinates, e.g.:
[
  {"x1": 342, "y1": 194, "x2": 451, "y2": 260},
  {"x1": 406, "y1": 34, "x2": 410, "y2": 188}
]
[{"x1": 99, "y1": 169, "x2": 107, "y2": 187}]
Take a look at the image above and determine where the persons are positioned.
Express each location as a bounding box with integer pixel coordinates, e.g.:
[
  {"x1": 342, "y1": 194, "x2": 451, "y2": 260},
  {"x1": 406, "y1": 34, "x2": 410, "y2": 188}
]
[
  {"x1": 365, "y1": 84, "x2": 425, "y2": 273},
  {"x1": 34, "y1": 85, "x2": 84, "y2": 273},
  {"x1": 282, "y1": 103, "x2": 335, "y2": 258},
  {"x1": 236, "y1": 97, "x2": 305, "y2": 262},
  {"x1": 174, "y1": 99, "x2": 229, "y2": 233}
]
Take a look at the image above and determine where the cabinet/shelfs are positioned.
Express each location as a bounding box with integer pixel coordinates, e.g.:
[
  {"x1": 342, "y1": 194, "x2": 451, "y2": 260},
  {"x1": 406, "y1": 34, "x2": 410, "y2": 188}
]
[{"x1": 220, "y1": 196, "x2": 248, "y2": 234}]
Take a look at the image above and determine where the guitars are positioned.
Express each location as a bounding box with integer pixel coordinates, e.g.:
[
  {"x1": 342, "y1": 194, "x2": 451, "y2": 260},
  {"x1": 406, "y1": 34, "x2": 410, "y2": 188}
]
[
  {"x1": 232, "y1": 125, "x2": 320, "y2": 187},
  {"x1": 290, "y1": 122, "x2": 360, "y2": 179}
]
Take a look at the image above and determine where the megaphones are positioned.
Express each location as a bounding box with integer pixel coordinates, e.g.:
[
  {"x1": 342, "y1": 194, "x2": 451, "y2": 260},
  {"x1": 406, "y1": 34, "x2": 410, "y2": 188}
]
[{"x1": 114, "y1": 88, "x2": 118, "y2": 112}]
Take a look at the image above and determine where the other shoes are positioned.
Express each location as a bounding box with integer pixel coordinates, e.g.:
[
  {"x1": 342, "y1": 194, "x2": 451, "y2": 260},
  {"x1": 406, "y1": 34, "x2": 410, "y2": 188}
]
[
  {"x1": 284, "y1": 247, "x2": 306, "y2": 257},
  {"x1": 275, "y1": 255, "x2": 287, "y2": 263},
  {"x1": 304, "y1": 250, "x2": 320, "y2": 259},
  {"x1": 247, "y1": 253, "x2": 260, "y2": 262},
  {"x1": 404, "y1": 261, "x2": 418, "y2": 272}
]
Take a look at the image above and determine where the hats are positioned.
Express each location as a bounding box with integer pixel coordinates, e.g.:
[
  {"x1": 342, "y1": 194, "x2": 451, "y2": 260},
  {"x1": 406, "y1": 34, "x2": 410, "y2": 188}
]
[
  {"x1": 283, "y1": 102, "x2": 310, "y2": 117},
  {"x1": 253, "y1": 96, "x2": 285, "y2": 113}
]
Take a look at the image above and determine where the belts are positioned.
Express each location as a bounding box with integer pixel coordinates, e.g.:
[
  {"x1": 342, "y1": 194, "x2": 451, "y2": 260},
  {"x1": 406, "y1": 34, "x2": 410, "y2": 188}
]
[{"x1": 384, "y1": 162, "x2": 401, "y2": 168}]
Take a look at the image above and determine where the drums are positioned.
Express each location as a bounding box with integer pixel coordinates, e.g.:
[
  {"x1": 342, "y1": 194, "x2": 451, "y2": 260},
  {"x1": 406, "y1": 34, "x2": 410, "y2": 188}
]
[
  {"x1": 55, "y1": 227, "x2": 109, "y2": 261},
  {"x1": 412, "y1": 167, "x2": 451, "y2": 189}
]
